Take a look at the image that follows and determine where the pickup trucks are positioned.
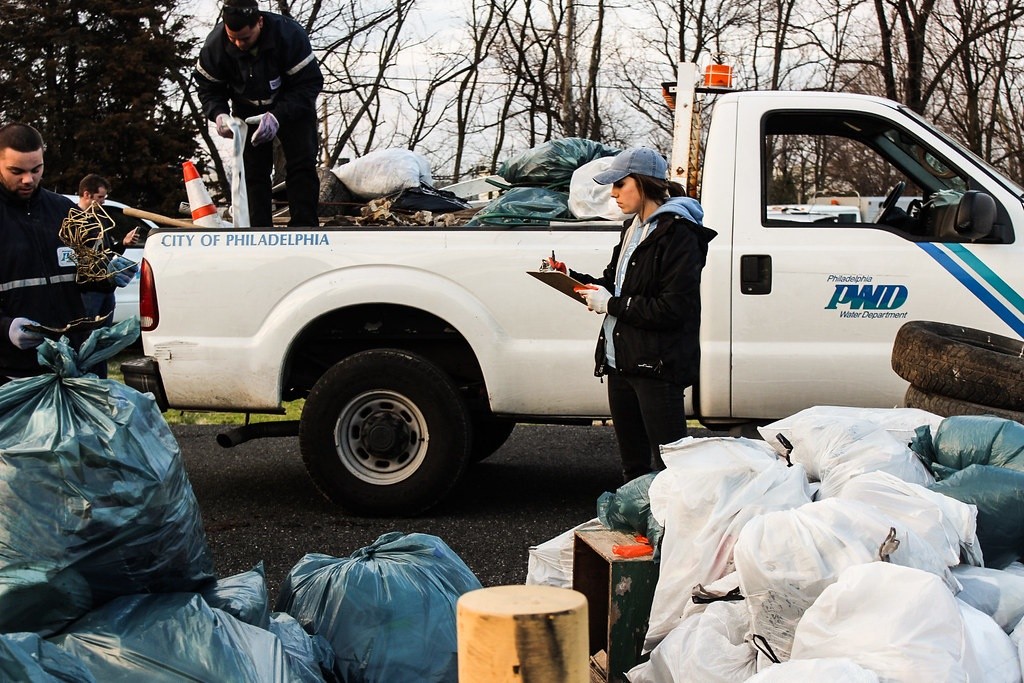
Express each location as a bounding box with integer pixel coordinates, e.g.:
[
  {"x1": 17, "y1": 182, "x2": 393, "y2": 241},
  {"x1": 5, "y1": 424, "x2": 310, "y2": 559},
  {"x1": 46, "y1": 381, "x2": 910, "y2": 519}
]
[{"x1": 138, "y1": 61, "x2": 1024, "y2": 522}]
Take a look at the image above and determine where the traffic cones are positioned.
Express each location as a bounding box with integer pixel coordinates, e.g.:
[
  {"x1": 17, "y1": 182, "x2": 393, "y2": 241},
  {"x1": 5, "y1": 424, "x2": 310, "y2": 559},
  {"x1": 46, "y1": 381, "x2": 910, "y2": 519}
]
[{"x1": 181, "y1": 161, "x2": 222, "y2": 228}]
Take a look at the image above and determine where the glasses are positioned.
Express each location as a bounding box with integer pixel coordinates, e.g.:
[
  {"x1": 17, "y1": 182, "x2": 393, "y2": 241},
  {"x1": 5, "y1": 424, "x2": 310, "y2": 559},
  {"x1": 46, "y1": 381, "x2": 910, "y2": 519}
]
[{"x1": 223, "y1": 4, "x2": 258, "y2": 18}]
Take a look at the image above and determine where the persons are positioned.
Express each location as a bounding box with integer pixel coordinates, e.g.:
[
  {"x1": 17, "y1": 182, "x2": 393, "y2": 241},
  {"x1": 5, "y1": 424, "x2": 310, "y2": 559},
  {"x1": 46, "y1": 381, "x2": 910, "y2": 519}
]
[
  {"x1": 74, "y1": 174, "x2": 140, "y2": 380},
  {"x1": 192, "y1": 0, "x2": 324, "y2": 228},
  {"x1": 0, "y1": 122, "x2": 138, "y2": 388},
  {"x1": 548, "y1": 146, "x2": 718, "y2": 482}
]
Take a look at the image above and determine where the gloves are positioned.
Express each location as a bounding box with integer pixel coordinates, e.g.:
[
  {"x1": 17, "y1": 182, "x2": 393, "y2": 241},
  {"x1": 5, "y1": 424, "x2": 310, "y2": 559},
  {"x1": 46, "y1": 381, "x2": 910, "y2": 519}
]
[
  {"x1": 573, "y1": 283, "x2": 613, "y2": 316},
  {"x1": 246, "y1": 112, "x2": 280, "y2": 148},
  {"x1": 215, "y1": 114, "x2": 233, "y2": 140},
  {"x1": 107, "y1": 256, "x2": 139, "y2": 287},
  {"x1": 548, "y1": 255, "x2": 570, "y2": 278},
  {"x1": 9, "y1": 317, "x2": 47, "y2": 349}
]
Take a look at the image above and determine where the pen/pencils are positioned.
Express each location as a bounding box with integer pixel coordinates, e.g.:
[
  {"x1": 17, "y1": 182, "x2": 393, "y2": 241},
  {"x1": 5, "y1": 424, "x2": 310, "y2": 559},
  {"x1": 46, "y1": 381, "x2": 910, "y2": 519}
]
[{"x1": 551, "y1": 249, "x2": 557, "y2": 271}]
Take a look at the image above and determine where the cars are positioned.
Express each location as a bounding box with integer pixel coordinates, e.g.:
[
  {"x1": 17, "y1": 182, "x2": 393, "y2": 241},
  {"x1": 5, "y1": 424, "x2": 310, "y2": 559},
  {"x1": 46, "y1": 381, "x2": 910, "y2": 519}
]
[{"x1": 62, "y1": 194, "x2": 161, "y2": 356}]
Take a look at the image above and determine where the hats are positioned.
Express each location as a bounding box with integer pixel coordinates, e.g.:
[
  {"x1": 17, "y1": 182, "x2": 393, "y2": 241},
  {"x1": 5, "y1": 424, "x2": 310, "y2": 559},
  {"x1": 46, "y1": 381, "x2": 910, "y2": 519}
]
[{"x1": 593, "y1": 146, "x2": 667, "y2": 185}]
